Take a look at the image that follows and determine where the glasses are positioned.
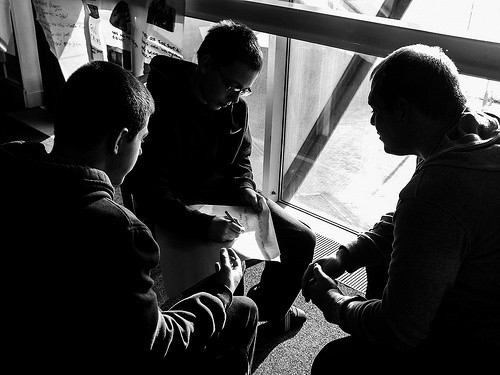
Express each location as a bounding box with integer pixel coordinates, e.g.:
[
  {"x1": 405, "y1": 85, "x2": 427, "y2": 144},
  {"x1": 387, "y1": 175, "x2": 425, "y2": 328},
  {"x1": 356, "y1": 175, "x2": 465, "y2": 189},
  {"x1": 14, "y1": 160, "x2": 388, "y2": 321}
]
[{"x1": 217, "y1": 67, "x2": 251, "y2": 97}]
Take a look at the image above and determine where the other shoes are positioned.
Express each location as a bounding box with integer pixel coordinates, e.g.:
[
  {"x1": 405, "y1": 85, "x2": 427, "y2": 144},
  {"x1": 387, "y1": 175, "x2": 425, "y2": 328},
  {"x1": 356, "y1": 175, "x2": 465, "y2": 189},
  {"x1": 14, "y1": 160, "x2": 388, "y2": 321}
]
[{"x1": 255, "y1": 301, "x2": 307, "y2": 331}]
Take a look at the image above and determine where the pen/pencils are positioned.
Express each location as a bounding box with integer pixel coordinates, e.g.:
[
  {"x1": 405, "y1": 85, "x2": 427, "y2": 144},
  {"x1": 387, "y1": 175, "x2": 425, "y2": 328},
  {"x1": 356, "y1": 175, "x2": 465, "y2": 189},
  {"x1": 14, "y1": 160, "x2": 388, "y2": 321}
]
[{"x1": 223, "y1": 210, "x2": 237, "y2": 226}]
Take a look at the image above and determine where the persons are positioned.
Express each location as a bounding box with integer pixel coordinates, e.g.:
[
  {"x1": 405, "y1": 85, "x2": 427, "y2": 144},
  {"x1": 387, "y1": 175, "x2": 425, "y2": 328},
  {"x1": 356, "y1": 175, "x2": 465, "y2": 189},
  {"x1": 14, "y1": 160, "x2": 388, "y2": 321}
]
[
  {"x1": 0, "y1": 61, "x2": 258, "y2": 375},
  {"x1": 132, "y1": 18, "x2": 315, "y2": 339},
  {"x1": 302, "y1": 43, "x2": 500, "y2": 374}
]
